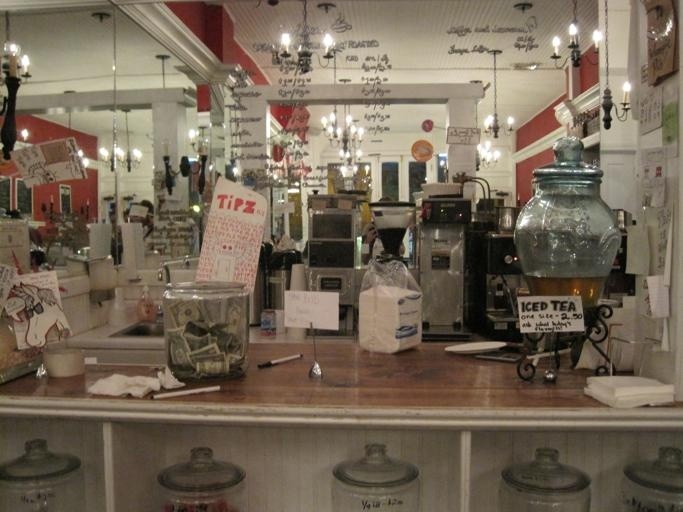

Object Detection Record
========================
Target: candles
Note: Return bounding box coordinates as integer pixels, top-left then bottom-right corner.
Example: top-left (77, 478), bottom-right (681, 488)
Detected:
top-left (9, 55), bottom-right (18, 77)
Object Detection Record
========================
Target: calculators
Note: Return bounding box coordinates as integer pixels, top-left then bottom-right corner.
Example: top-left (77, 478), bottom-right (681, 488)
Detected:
top-left (476, 351), bottom-right (521, 363)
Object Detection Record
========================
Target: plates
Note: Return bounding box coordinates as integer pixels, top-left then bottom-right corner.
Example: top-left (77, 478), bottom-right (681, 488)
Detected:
top-left (444, 342), bottom-right (507, 355)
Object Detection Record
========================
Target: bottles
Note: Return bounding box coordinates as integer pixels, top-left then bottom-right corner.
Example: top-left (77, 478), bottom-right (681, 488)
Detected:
top-left (623, 445), bottom-right (683, 512)
top-left (501, 447), bottom-right (594, 512)
top-left (153, 445), bottom-right (247, 512)
top-left (332, 442), bottom-right (422, 512)
top-left (163, 280), bottom-right (249, 383)
top-left (0, 440), bottom-right (88, 511)
top-left (513, 135), bottom-right (622, 312)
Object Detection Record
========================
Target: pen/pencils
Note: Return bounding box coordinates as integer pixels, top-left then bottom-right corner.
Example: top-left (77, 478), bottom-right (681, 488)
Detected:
top-left (150, 384), bottom-right (225, 400)
top-left (257, 353), bottom-right (303, 368)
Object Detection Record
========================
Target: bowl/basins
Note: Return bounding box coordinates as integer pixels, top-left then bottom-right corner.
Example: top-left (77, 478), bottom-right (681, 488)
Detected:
top-left (42, 350), bottom-right (87, 379)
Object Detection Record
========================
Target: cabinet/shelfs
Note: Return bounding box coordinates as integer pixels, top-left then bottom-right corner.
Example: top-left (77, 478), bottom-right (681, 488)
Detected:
top-left (0, 341), bottom-right (683, 511)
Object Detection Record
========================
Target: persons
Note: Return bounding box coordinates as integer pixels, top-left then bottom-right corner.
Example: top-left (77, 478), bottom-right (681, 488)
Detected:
top-left (359, 196), bottom-right (406, 264)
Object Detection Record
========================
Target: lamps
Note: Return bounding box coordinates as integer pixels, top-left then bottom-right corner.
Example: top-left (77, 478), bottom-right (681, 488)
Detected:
top-left (271, 1), bottom-right (335, 74)
top-left (1, 10), bottom-right (33, 85)
top-left (550, 0), bottom-right (605, 71)
top-left (320, 102), bottom-right (365, 168)
top-left (471, 48), bottom-right (515, 168)
top-left (600, 0), bottom-right (633, 130)
top-left (181, 126), bottom-right (220, 193)
top-left (99, 107), bottom-right (140, 172)
